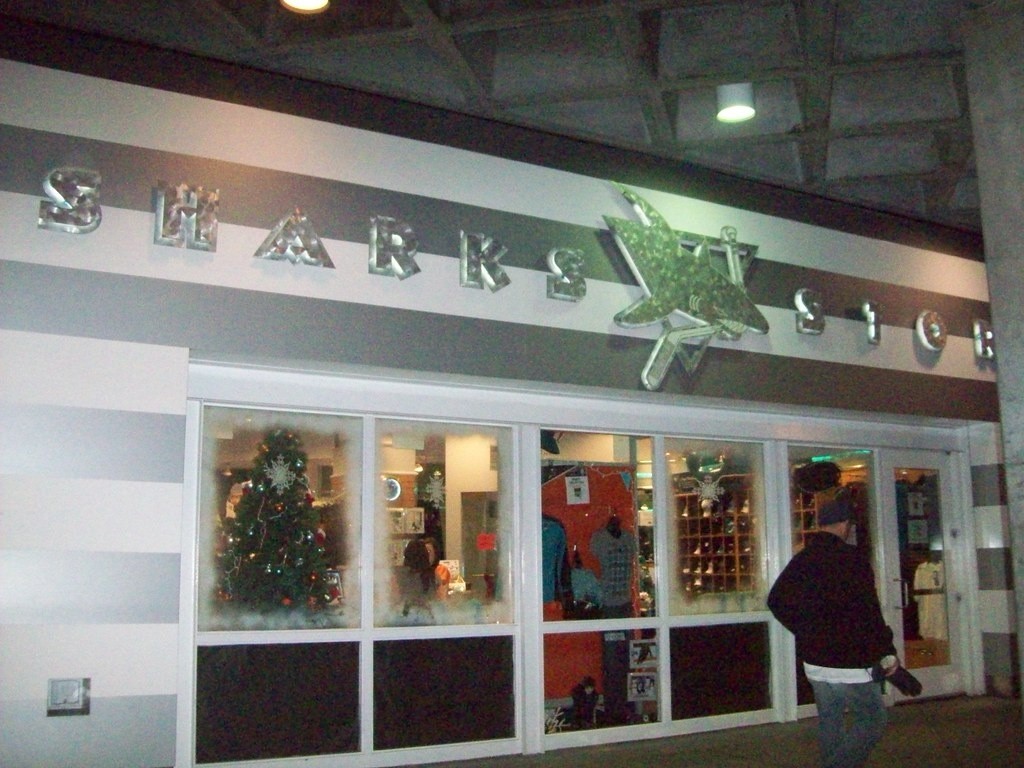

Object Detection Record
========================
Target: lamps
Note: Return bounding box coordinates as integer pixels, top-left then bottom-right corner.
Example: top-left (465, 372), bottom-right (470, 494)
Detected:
top-left (415, 464), bottom-right (423, 472)
top-left (717, 81), bottom-right (757, 123)
top-left (324, 570), bottom-right (346, 598)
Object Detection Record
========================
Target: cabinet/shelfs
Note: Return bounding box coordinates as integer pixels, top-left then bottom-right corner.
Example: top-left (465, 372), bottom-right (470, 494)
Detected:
top-left (675, 483), bottom-right (843, 608)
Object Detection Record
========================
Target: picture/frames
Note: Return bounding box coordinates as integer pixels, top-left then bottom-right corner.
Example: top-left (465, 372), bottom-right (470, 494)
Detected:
top-left (406, 508), bottom-right (425, 534)
top-left (907, 492), bottom-right (925, 516)
top-left (630, 639), bottom-right (657, 669)
top-left (907, 520), bottom-right (929, 543)
top-left (385, 508), bottom-right (405, 534)
top-left (387, 539), bottom-right (405, 567)
top-left (383, 477), bottom-right (403, 502)
top-left (627, 672), bottom-right (657, 702)
top-left (564, 476), bottom-right (590, 505)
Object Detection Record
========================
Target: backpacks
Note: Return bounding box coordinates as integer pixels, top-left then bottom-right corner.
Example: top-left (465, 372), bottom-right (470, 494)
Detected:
top-left (767, 543), bottom-right (851, 639)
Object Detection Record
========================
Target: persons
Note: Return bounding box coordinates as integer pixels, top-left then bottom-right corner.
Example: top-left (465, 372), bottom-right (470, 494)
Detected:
top-left (590, 514), bottom-right (638, 712)
top-left (912, 544), bottom-right (952, 663)
top-left (399, 538), bottom-right (450, 614)
top-left (766, 498), bottom-right (901, 768)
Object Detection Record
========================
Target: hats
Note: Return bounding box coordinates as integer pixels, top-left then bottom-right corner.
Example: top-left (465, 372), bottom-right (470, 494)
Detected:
top-left (816, 501), bottom-right (848, 525)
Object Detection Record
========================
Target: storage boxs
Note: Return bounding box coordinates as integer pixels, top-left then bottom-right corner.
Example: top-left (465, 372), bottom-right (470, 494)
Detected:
top-left (439, 559), bottom-right (466, 592)
top-left (638, 510), bottom-right (653, 527)
